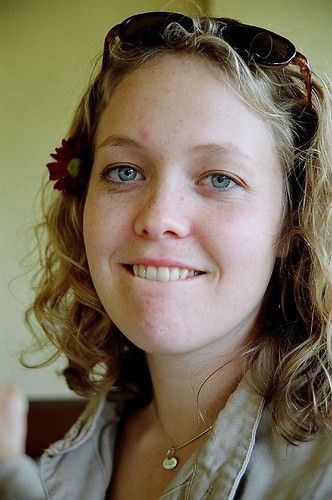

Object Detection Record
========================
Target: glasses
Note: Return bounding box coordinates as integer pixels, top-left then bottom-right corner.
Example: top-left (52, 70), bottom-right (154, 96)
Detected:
top-left (103, 10), bottom-right (313, 120)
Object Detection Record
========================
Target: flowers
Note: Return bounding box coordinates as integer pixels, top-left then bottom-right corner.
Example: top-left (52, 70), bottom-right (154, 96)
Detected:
top-left (47, 136), bottom-right (92, 197)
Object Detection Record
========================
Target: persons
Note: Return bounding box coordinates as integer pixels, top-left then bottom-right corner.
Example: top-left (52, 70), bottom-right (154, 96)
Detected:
top-left (1, 11), bottom-right (331, 497)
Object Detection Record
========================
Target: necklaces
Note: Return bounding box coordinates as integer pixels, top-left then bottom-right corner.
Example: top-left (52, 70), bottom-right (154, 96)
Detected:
top-left (146, 386), bottom-right (222, 471)
